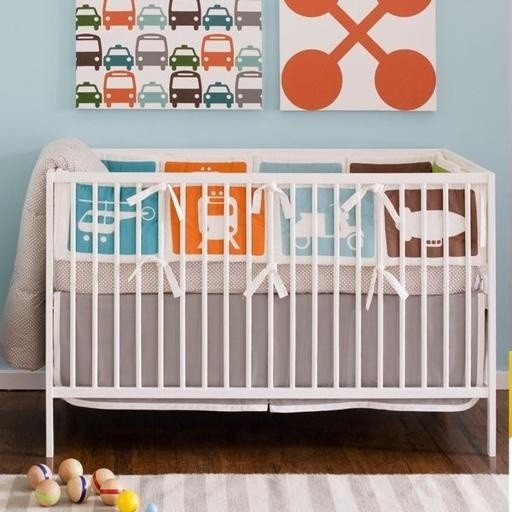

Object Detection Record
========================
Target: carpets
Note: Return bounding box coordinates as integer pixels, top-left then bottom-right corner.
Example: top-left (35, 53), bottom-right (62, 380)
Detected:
top-left (0, 473), bottom-right (510, 512)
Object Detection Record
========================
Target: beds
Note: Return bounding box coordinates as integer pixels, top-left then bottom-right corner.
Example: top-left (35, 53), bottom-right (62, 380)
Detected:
top-left (44, 146), bottom-right (496, 458)
